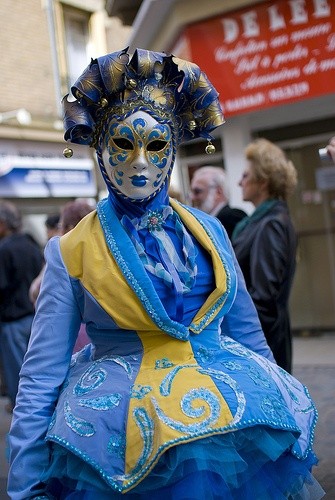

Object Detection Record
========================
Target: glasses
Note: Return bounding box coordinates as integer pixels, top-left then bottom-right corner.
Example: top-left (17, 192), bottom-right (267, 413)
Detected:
top-left (194, 182), bottom-right (217, 195)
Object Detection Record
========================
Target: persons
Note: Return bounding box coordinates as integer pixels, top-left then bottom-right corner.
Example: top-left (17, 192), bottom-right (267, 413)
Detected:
top-left (0, 199), bottom-right (95, 414)
top-left (231, 137), bottom-right (299, 374)
top-left (7, 45), bottom-right (326, 500)
top-left (189, 165), bottom-right (248, 240)
